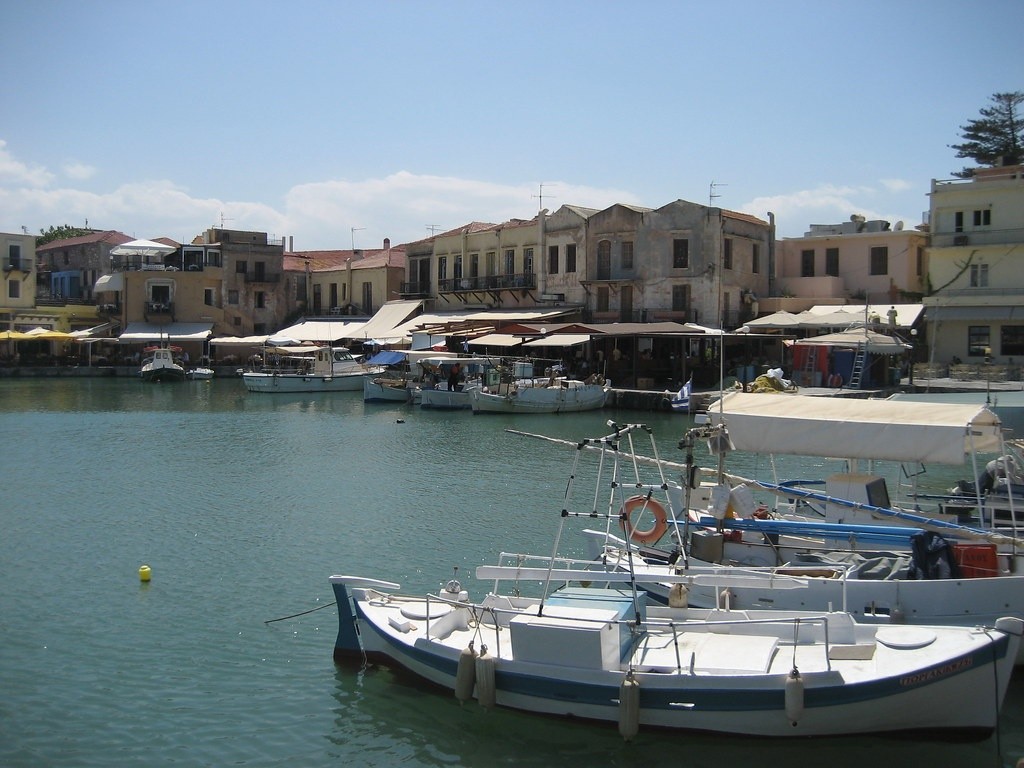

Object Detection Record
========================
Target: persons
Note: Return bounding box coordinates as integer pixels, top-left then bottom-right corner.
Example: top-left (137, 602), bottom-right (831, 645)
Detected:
top-left (642, 348), bottom-right (655, 361)
top-left (596, 345), bottom-right (622, 374)
top-left (102, 345), bottom-right (140, 365)
top-left (886, 305), bottom-right (897, 331)
top-left (868, 311), bottom-right (881, 333)
top-left (173, 351), bottom-right (190, 367)
top-left (448, 363), bottom-right (461, 392)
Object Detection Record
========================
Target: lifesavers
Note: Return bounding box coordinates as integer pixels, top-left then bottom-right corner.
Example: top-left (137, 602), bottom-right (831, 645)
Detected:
top-left (619, 495), bottom-right (667, 543)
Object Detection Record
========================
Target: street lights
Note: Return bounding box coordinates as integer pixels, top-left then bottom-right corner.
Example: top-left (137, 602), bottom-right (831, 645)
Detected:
top-left (909, 328), bottom-right (918, 383)
top-left (984, 347), bottom-right (992, 404)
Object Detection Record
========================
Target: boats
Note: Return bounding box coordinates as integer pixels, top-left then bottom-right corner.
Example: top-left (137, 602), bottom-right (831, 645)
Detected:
top-left (136, 327), bottom-right (184, 383)
top-left (185, 368), bottom-right (215, 380)
top-left (240, 341), bottom-right (613, 416)
top-left (325, 416), bottom-right (1024, 742)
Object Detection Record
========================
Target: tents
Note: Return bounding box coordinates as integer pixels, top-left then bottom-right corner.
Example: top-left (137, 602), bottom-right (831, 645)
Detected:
top-left (208, 335), bottom-right (269, 372)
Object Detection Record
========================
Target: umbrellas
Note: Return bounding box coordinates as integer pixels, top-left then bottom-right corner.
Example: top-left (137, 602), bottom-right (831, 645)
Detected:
top-left (110, 239), bottom-right (177, 274)
top-left (0, 326), bottom-right (79, 367)
top-left (743, 306), bottom-right (901, 366)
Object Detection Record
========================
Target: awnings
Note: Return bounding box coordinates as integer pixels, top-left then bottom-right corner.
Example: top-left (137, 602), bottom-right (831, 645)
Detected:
top-left (521, 334), bottom-right (590, 348)
top-left (465, 307), bottom-right (580, 321)
top-left (810, 304), bottom-right (927, 331)
top-left (416, 356), bottom-right (501, 366)
top-left (93, 272), bottom-right (124, 293)
top-left (460, 333), bottom-right (533, 347)
top-left (362, 350), bottom-right (408, 366)
top-left (76, 337), bottom-right (118, 344)
top-left (518, 322), bottom-right (705, 336)
top-left (118, 322), bottom-right (215, 342)
top-left (265, 298), bottom-right (485, 345)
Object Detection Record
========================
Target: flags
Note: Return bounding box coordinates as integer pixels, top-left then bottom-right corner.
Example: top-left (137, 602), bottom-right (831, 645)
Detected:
top-left (671, 377), bottom-right (691, 411)
